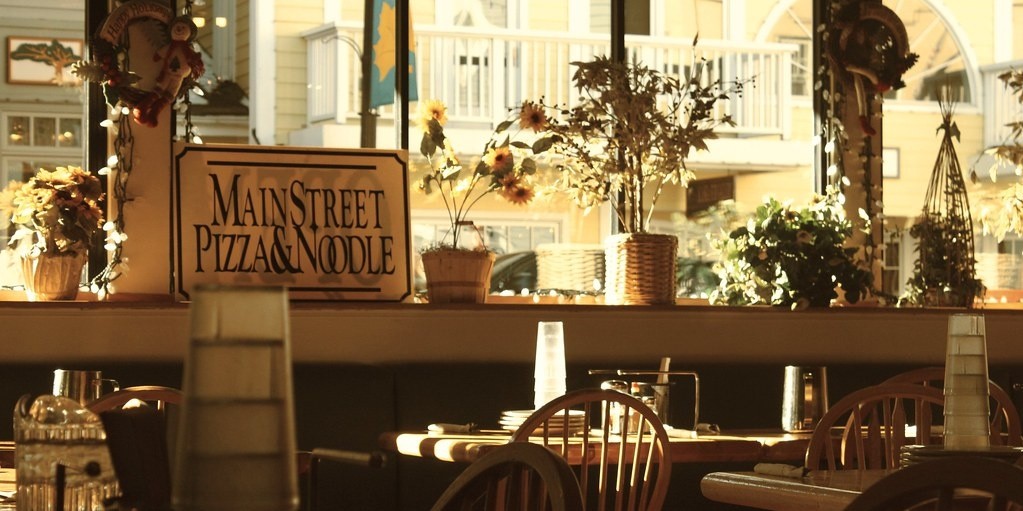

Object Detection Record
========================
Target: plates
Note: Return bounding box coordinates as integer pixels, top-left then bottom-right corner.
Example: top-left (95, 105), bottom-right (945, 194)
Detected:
top-left (498, 410), bottom-right (586, 437)
top-left (897, 444), bottom-right (1023, 471)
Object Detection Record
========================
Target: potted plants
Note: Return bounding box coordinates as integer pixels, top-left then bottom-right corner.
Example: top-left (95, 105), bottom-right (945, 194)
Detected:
top-left (533, 32), bottom-right (756, 304)
top-left (898, 89), bottom-right (987, 307)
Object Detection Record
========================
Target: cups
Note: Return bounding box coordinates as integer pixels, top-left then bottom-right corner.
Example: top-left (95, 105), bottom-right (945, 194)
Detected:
top-left (52, 368), bottom-right (120, 403)
top-left (532, 321), bottom-right (567, 410)
top-left (780, 365), bottom-right (828, 433)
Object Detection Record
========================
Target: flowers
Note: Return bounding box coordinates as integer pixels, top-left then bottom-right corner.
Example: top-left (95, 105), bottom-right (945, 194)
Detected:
top-left (3, 165), bottom-right (106, 253)
top-left (419, 101), bottom-right (549, 248)
top-left (708, 194), bottom-right (891, 311)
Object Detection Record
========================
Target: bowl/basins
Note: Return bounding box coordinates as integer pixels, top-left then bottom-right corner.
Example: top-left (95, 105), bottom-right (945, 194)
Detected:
top-left (942, 310), bottom-right (991, 449)
top-left (600, 380), bottom-right (670, 433)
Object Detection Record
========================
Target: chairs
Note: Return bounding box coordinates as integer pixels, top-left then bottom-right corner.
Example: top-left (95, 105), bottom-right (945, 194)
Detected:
top-left (841, 366), bottom-right (1020, 469)
top-left (804, 387), bottom-right (943, 470)
top-left (429, 441), bottom-right (582, 511)
top-left (86, 386), bottom-right (182, 415)
top-left (497, 389), bottom-right (670, 511)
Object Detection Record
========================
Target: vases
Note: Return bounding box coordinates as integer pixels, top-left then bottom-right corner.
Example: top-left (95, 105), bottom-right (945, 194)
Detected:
top-left (18, 255), bottom-right (90, 299)
top-left (420, 250), bottom-right (494, 305)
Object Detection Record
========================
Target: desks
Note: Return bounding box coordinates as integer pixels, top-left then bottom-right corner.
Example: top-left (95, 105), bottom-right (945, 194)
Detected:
top-left (765, 427), bottom-right (945, 459)
top-left (375, 432), bottom-right (761, 467)
top-left (701, 472), bottom-right (991, 511)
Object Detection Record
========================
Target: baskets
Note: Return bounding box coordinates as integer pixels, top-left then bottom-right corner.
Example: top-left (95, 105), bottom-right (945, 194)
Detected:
top-left (603, 234), bottom-right (677, 304)
